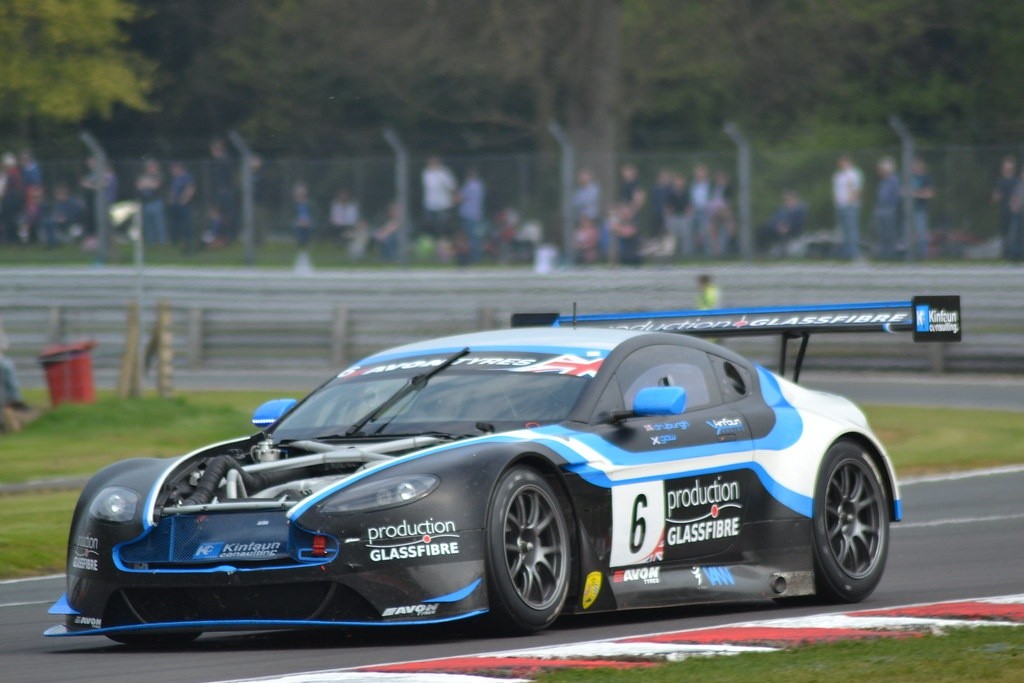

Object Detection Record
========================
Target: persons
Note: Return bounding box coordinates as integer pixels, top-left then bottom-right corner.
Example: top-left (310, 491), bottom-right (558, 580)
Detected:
top-left (759, 188), bottom-right (809, 258)
top-left (870, 155), bottom-right (901, 257)
top-left (906, 156), bottom-right (936, 264)
top-left (697, 273), bottom-right (721, 344)
top-left (0, 334), bottom-right (42, 432)
top-left (421, 152), bottom-right (458, 233)
top-left (834, 156), bottom-right (864, 258)
top-left (572, 161), bottom-right (737, 267)
top-left (0, 136), bottom-right (400, 267)
top-left (994, 157), bottom-right (1024, 267)
top-left (451, 161), bottom-right (485, 265)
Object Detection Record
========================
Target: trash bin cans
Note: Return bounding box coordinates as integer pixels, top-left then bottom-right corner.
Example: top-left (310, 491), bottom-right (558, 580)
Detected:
top-left (41, 340), bottom-right (95, 409)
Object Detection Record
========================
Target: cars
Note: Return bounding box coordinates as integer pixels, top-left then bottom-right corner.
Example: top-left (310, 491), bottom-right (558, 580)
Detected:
top-left (41, 294), bottom-right (962, 647)
